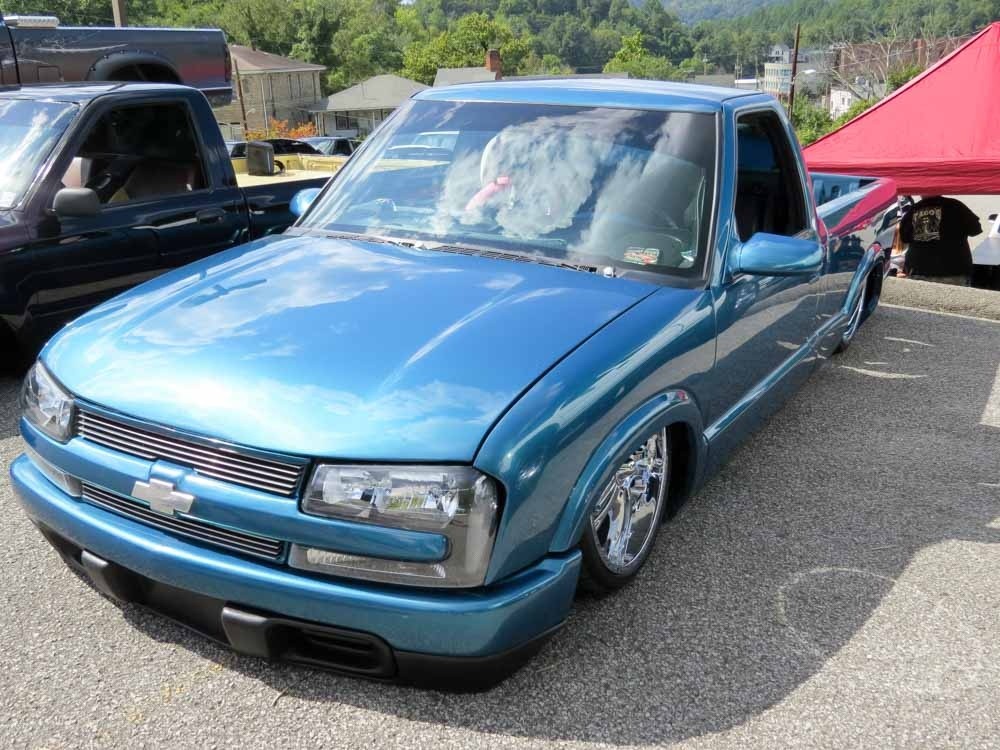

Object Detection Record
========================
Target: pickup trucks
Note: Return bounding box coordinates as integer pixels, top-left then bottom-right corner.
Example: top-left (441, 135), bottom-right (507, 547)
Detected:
top-left (0, 80), bottom-right (455, 388)
top-left (0, 10), bottom-right (238, 117)
top-left (8, 73), bottom-right (903, 694)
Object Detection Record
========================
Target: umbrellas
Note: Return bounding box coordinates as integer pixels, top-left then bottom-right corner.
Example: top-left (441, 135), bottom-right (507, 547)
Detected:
top-left (801, 19), bottom-right (1000, 241)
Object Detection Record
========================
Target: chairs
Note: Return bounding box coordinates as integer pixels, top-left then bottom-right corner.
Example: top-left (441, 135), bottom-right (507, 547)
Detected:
top-left (104, 133), bottom-right (200, 203)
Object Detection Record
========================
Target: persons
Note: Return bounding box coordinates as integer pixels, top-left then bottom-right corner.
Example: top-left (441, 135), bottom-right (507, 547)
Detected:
top-left (621, 157), bottom-right (689, 232)
top-left (84, 119), bottom-right (164, 208)
top-left (898, 191), bottom-right (983, 289)
top-left (458, 134), bottom-right (559, 226)
top-left (827, 233), bottom-right (864, 272)
